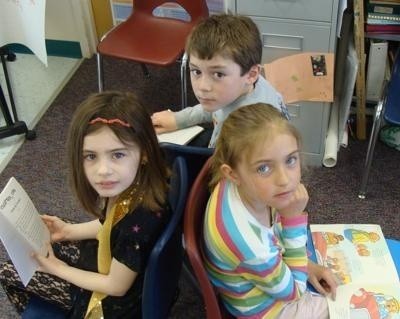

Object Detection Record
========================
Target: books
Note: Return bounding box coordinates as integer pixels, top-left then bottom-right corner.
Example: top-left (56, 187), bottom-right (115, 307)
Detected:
top-left (310, 224), bottom-right (400, 319)
top-left (0, 177), bottom-right (50, 287)
top-left (156, 109), bottom-right (204, 145)
top-left (366, 12), bottom-right (400, 35)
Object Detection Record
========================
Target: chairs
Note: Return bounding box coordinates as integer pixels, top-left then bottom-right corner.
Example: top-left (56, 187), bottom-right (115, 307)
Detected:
top-left (155, 143), bottom-right (217, 213)
top-left (183, 156), bottom-right (232, 318)
top-left (23, 156), bottom-right (188, 317)
top-left (96, 1), bottom-right (210, 115)
top-left (357, 69), bottom-right (400, 200)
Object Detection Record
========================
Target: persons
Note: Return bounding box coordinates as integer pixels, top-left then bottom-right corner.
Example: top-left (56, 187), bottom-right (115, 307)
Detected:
top-left (150, 14), bottom-right (290, 149)
top-left (202, 103), bottom-right (337, 319)
top-left (0, 92), bottom-right (175, 319)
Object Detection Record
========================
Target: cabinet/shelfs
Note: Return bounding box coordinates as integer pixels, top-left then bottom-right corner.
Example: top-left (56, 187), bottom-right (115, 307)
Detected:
top-left (230, 2), bottom-right (339, 168)
